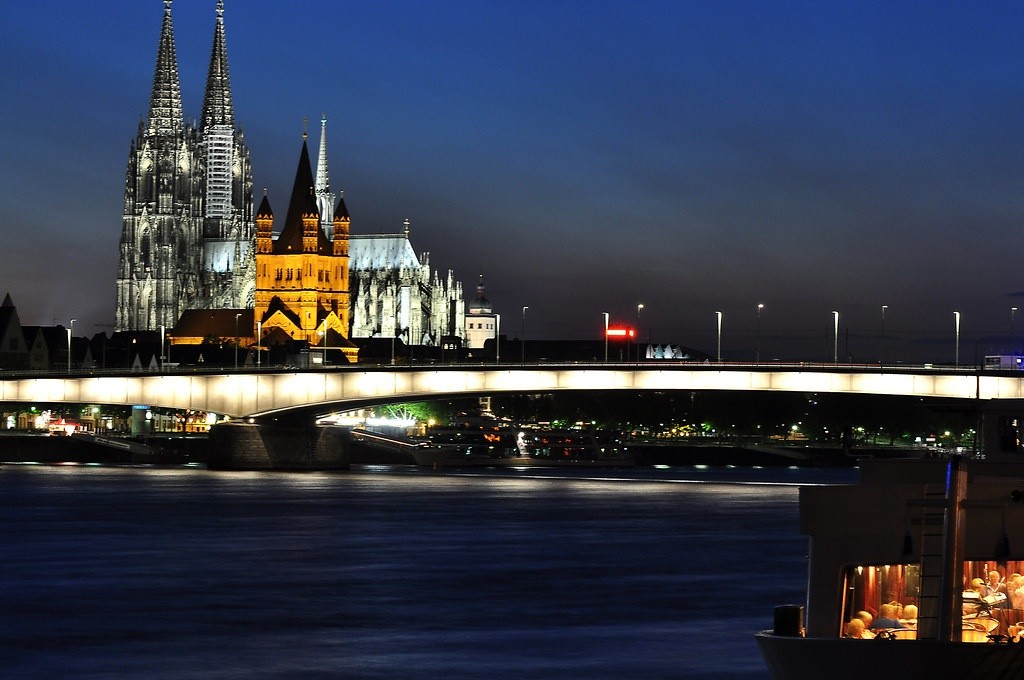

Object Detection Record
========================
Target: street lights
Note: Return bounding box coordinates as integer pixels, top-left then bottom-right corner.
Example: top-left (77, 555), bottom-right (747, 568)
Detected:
top-left (320, 318), bottom-right (328, 366)
top-left (494, 313), bottom-right (500, 364)
top-left (521, 306), bottom-right (530, 364)
top-left (256, 320), bottom-right (263, 366)
top-left (953, 311), bottom-right (960, 367)
top-left (65, 328), bottom-right (71, 372)
top-left (1010, 307), bottom-right (1017, 356)
top-left (234, 313), bottom-right (243, 368)
top-left (755, 304), bottom-right (764, 365)
top-left (158, 324), bottom-right (165, 372)
top-left (601, 312), bottom-right (609, 362)
top-left (636, 304), bottom-right (644, 369)
top-left (831, 310), bottom-right (839, 364)
top-left (715, 311), bottom-right (722, 363)
top-left (880, 305), bottom-right (888, 336)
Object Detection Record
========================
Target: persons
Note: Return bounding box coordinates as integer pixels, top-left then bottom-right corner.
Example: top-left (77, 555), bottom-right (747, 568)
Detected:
top-left (961, 570), bottom-right (1024, 643)
top-left (841, 600), bottom-right (918, 640)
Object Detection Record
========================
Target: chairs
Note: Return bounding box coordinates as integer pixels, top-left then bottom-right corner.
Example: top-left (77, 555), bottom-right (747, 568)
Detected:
top-left (870, 614), bottom-right (1000, 643)
